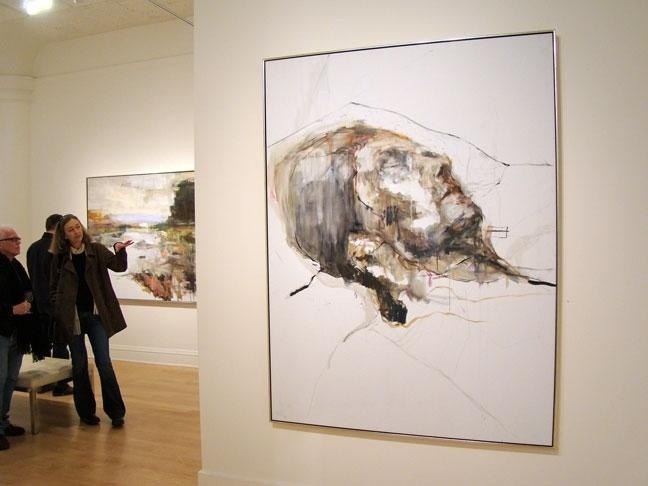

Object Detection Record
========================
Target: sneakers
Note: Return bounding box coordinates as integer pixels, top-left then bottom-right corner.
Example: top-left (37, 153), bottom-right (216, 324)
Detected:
top-left (0, 434), bottom-right (9, 451)
top-left (1, 420), bottom-right (26, 436)
top-left (51, 383), bottom-right (73, 397)
top-left (38, 383), bottom-right (54, 393)
top-left (79, 414), bottom-right (101, 423)
top-left (111, 419), bottom-right (124, 426)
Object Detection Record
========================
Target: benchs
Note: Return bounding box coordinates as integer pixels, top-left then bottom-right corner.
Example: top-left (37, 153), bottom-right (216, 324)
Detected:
top-left (15, 353), bottom-right (95, 434)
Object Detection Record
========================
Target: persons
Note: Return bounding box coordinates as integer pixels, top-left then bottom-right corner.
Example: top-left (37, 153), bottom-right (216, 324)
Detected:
top-left (0, 226), bottom-right (30, 450)
top-left (274, 124), bottom-right (553, 444)
top-left (45, 215), bottom-right (134, 426)
top-left (27, 213), bottom-right (75, 396)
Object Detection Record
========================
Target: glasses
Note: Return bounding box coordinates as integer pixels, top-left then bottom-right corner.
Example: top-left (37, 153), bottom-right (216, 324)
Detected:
top-left (0, 236), bottom-right (21, 242)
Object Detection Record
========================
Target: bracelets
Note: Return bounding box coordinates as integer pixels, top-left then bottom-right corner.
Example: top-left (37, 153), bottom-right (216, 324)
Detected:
top-left (114, 242), bottom-right (122, 247)
